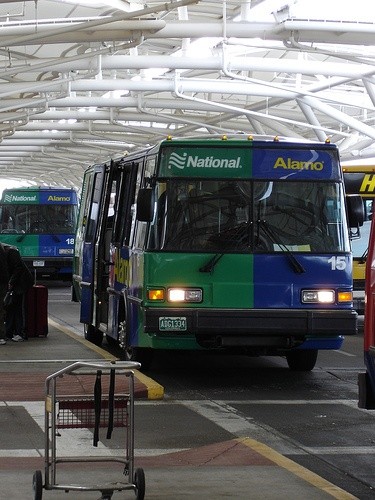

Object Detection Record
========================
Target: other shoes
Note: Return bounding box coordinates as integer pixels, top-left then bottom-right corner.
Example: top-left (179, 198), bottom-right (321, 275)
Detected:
top-left (12, 335), bottom-right (24, 342)
top-left (0, 339), bottom-right (6, 345)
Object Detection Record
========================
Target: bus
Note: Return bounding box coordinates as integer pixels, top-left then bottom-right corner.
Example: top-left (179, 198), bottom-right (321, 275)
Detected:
top-left (0, 186), bottom-right (80, 283)
top-left (70, 134), bottom-right (360, 384)
top-left (358, 191), bottom-right (375, 412)
top-left (337, 164), bottom-right (375, 313)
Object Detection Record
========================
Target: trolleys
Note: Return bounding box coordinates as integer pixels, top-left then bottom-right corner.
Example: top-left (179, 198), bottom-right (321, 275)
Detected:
top-left (32, 360), bottom-right (146, 500)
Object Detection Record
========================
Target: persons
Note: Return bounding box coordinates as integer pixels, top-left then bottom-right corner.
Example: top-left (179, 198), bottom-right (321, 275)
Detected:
top-left (0, 242), bottom-right (35, 345)
